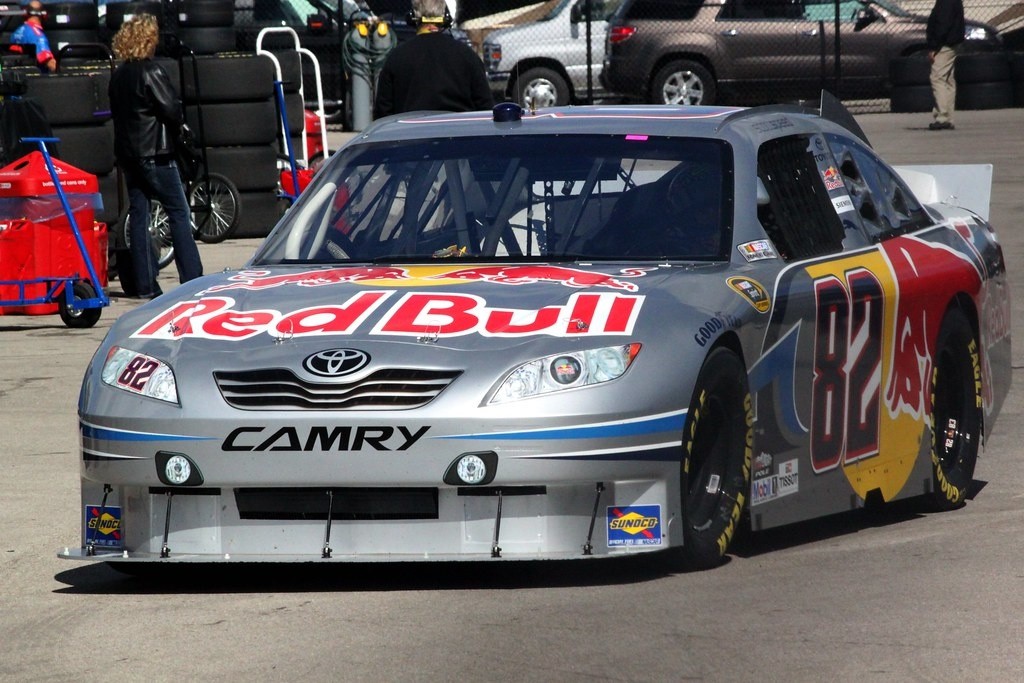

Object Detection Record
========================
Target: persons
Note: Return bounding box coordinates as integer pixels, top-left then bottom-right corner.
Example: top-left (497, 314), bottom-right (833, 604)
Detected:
top-left (926, 0), bottom-right (966, 130)
top-left (373, 0), bottom-right (496, 122)
top-left (110, 13), bottom-right (204, 299)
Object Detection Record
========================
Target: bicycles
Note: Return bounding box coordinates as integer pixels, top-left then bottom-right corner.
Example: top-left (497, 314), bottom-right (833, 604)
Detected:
top-left (119, 123), bottom-right (242, 270)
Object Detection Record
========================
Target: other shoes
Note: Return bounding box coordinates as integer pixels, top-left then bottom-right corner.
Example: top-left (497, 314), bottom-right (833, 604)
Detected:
top-left (929, 121), bottom-right (952, 130)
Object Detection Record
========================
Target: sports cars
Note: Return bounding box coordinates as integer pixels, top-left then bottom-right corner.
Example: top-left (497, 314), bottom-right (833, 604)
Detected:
top-left (58, 90), bottom-right (1013, 578)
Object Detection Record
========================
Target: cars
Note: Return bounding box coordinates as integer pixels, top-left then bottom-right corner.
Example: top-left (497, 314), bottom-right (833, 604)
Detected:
top-left (484, 0), bottom-right (612, 107)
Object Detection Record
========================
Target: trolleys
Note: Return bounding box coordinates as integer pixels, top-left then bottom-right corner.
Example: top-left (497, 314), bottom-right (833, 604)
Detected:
top-left (257, 27), bottom-right (352, 236)
top-left (0, 138), bottom-right (108, 328)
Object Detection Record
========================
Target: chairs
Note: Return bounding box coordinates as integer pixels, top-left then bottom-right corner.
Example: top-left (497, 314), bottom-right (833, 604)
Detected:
top-left (575, 157), bottom-right (722, 256)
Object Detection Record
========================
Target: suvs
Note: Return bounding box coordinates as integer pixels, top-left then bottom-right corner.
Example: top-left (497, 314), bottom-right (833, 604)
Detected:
top-left (99, 0), bottom-right (471, 123)
top-left (598, 0), bottom-right (1005, 106)
